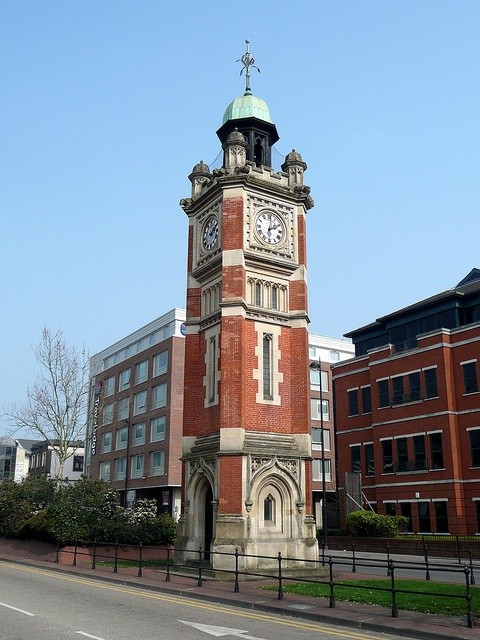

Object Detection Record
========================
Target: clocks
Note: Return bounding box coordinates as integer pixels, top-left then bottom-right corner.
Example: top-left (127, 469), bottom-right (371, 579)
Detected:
top-left (197, 210), bottom-right (221, 254)
top-left (253, 209), bottom-right (293, 251)
